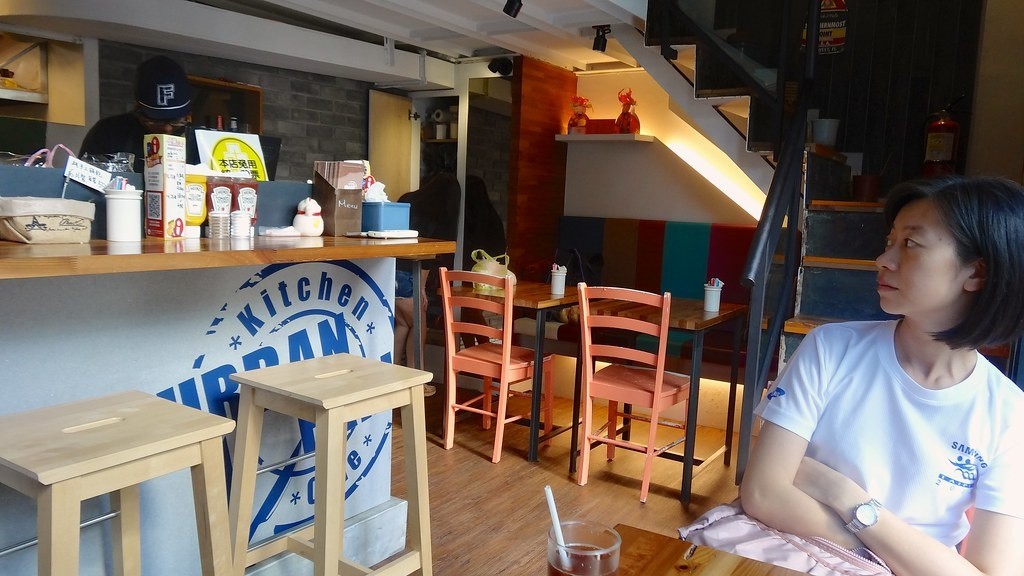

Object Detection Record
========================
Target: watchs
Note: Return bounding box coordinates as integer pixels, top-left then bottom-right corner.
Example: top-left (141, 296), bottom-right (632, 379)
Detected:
top-left (844, 498), bottom-right (883, 535)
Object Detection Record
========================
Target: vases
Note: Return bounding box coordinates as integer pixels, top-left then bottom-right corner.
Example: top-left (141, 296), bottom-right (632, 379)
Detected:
top-left (811, 118), bottom-right (840, 147)
top-left (852, 174), bottom-right (881, 203)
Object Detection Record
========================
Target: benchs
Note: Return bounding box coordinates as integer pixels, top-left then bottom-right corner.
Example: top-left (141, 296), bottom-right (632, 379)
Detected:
top-left (515, 309), bottom-right (779, 387)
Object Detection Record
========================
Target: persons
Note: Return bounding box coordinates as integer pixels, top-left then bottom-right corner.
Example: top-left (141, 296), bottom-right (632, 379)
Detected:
top-left (76, 61), bottom-right (217, 175)
top-left (388, 146), bottom-right (506, 394)
top-left (740, 174), bottom-right (1024, 576)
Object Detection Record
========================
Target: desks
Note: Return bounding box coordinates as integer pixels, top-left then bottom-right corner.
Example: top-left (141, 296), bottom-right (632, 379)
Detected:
top-left (437, 281), bottom-right (583, 463)
top-left (568, 298), bottom-right (747, 505)
top-left (603, 523), bottom-right (816, 576)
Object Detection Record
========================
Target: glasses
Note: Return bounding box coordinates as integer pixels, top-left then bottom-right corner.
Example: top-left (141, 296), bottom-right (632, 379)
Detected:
top-left (147, 111), bottom-right (188, 127)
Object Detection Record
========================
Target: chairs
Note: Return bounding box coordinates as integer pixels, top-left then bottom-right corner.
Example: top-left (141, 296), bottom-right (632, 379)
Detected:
top-left (577, 282), bottom-right (691, 503)
top-left (439, 267), bottom-right (555, 464)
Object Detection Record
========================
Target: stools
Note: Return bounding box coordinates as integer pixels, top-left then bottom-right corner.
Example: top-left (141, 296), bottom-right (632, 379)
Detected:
top-left (0, 390), bottom-right (236, 576)
top-left (229, 352), bottom-right (433, 576)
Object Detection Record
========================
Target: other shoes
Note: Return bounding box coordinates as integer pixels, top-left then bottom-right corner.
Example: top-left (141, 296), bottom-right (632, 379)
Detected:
top-left (423, 384), bottom-right (436, 397)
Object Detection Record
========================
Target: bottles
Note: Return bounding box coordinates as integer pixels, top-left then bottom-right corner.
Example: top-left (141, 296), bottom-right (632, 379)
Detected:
top-left (231, 117), bottom-right (238, 132)
top-left (217, 115), bottom-right (224, 131)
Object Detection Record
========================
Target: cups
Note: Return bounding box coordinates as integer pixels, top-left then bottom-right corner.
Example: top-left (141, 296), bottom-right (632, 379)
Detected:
top-left (209, 212), bottom-right (230, 240)
top-left (450, 119), bottom-right (458, 138)
top-left (703, 284), bottom-right (722, 312)
top-left (231, 212), bottom-right (252, 239)
top-left (436, 122), bottom-right (448, 139)
top-left (105, 189), bottom-right (144, 242)
top-left (239, 123), bottom-right (252, 133)
top-left (546, 521), bottom-right (621, 576)
top-left (551, 270), bottom-right (567, 295)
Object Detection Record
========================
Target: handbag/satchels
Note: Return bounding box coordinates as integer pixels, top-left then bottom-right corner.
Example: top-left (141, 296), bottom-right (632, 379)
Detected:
top-left (471, 249), bottom-right (518, 288)
top-left (677, 486), bottom-right (899, 576)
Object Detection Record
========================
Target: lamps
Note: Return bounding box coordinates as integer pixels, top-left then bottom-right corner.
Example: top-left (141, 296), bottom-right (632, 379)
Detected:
top-left (661, 44), bottom-right (677, 61)
top-left (503, 0), bottom-right (523, 18)
top-left (488, 58), bottom-right (514, 75)
top-left (592, 24), bottom-right (611, 53)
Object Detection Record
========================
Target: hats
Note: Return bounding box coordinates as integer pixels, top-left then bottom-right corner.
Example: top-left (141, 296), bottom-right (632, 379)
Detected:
top-left (134, 56), bottom-right (195, 121)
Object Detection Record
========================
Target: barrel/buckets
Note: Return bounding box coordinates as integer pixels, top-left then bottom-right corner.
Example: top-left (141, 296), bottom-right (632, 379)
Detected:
top-left (811, 118), bottom-right (839, 146)
top-left (853, 175), bottom-right (880, 202)
